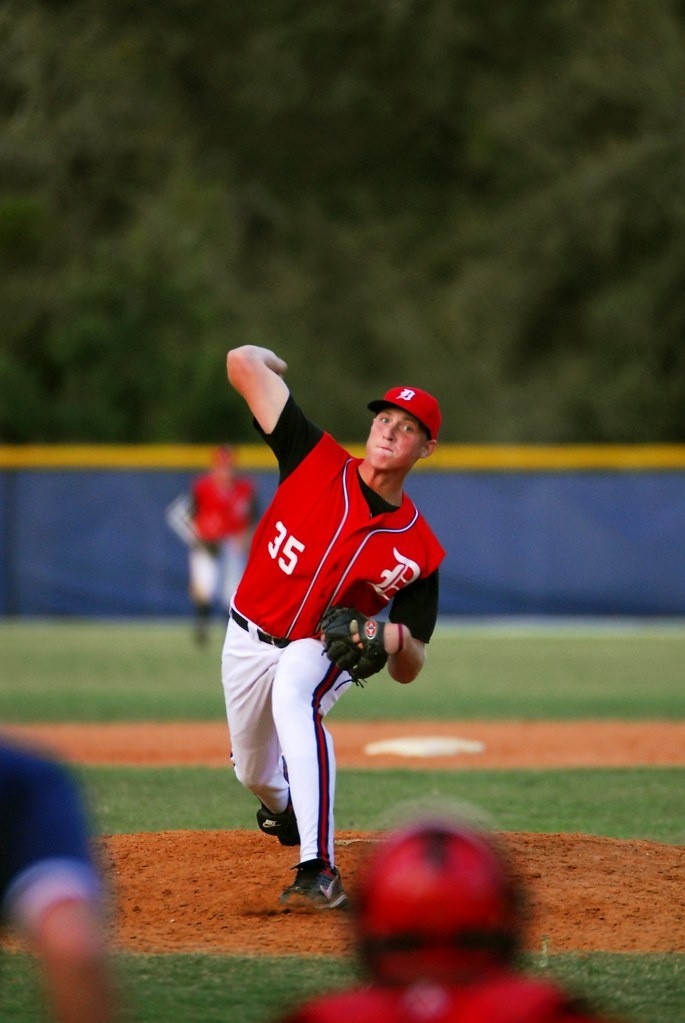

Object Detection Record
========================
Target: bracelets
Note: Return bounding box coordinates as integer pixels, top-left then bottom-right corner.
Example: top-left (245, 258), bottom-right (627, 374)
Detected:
top-left (391, 622), bottom-right (403, 659)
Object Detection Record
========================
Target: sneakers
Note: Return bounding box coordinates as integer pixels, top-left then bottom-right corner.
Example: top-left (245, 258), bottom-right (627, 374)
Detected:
top-left (279, 858), bottom-right (348, 909)
top-left (256, 756), bottom-right (300, 846)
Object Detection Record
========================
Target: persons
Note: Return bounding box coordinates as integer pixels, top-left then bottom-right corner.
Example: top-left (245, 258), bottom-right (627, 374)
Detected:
top-left (167, 444), bottom-right (259, 644)
top-left (284, 795), bottom-right (605, 1023)
top-left (221, 344), bottom-right (447, 908)
top-left (0, 744), bottom-right (116, 1023)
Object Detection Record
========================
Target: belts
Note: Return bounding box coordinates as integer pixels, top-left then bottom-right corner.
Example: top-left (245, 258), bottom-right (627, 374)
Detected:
top-left (231, 609), bottom-right (292, 649)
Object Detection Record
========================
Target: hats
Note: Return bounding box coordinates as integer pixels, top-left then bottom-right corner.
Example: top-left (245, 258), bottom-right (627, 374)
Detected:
top-left (368, 386), bottom-right (441, 440)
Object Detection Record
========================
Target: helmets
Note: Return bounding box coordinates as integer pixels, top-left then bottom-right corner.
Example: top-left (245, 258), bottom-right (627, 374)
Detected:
top-left (356, 821), bottom-right (511, 985)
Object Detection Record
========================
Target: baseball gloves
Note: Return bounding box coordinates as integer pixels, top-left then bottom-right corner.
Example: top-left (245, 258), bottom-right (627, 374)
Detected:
top-left (317, 605), bottom-right (388, 692)
